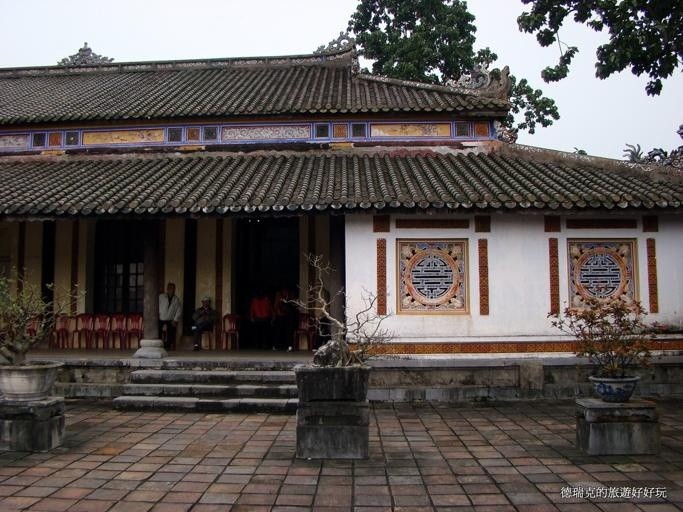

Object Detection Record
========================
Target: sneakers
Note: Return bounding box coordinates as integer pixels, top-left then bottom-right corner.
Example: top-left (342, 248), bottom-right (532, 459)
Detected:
top-left (194, 346), bottom-right (199, 352)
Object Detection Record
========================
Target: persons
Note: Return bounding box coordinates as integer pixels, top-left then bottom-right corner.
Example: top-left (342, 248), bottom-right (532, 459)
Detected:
top-left (312, 278), bottom-right (331, 354)
top-left (271, 286), bottom-right (297, 353)
top-left (190, 296), bottom-right (216, 352)
top-left (158, 281), bottom-right (182, 352)
top-left (249, 287), bottom-right (276, 353)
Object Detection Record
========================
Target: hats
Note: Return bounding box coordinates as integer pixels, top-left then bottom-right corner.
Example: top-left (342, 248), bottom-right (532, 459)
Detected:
top-left (202, 297), bottom-right (210, 301)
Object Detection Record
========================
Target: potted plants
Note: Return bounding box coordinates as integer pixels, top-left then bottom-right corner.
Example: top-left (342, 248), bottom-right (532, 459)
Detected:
top-left (0, 266), bottom-right (85, 400)
top-left (547, 292), bottom-right (667, 403)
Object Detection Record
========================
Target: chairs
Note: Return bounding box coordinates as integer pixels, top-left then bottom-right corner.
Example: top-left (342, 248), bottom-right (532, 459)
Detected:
top-left (293, 313), bottom-right (314, 351)
top-left (22, 313), bottom-right (241, 351)
